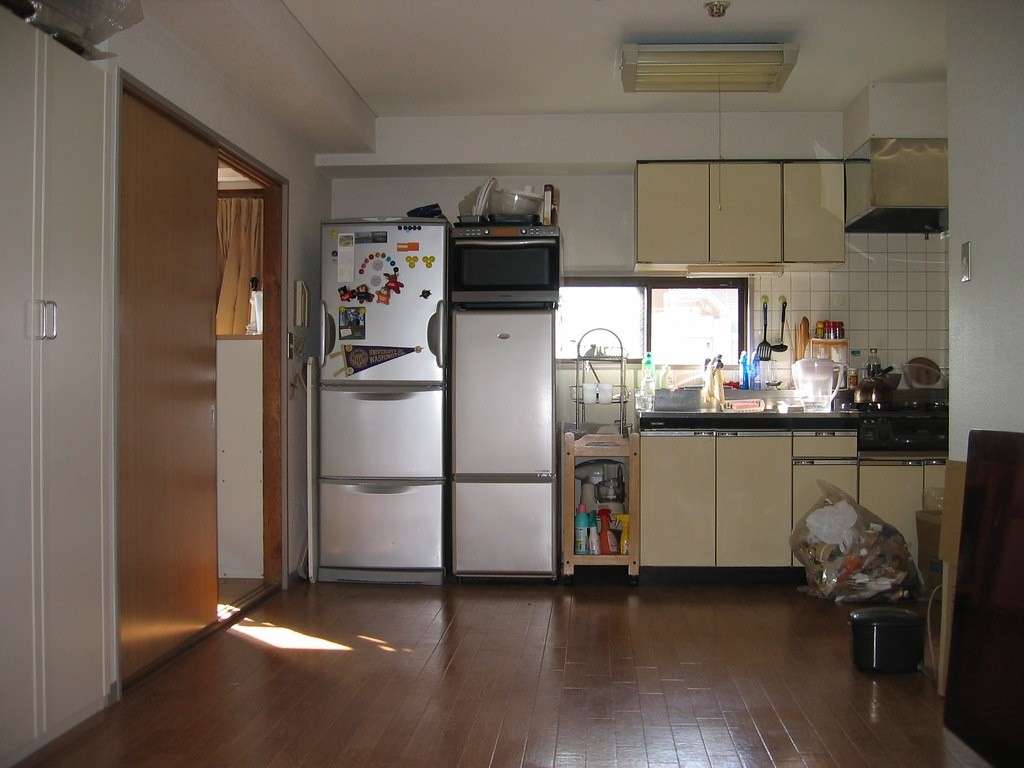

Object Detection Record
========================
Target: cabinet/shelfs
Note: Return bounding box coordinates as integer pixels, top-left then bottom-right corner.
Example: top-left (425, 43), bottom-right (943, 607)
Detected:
top-left (563, 328), bottom-right (640, 587)
top-left (633, 159), bottom-right (846, 272)
top-left (0, 5), bottom-right (120, 768)
top-left (639, 428), bottom-right (947, 588)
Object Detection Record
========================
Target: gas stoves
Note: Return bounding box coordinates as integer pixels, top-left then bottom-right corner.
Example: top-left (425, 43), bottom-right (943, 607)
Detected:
top-left (832, 387), bottom-right (949, 452)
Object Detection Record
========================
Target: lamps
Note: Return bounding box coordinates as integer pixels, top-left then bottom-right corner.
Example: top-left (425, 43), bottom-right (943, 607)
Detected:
top-left (618, 42), bottom-right (800, 92)
top-left (684, 267), bottom-right (784, 278)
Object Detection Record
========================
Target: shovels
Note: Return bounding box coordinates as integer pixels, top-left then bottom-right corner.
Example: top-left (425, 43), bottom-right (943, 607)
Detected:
top-left (756, 302), bottom-right (772, 361)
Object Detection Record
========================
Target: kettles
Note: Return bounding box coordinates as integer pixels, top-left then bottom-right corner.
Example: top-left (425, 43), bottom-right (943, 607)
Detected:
top-left (853, 366), bottom-right (895, 404)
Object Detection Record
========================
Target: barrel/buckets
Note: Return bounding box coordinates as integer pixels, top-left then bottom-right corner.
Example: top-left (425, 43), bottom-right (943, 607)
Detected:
top-left (849, 606), bottom-right (926, 674)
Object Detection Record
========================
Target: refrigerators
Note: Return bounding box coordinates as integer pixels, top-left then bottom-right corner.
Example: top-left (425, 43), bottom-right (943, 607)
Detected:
top-left (313, 216), bottom-right (450, 587)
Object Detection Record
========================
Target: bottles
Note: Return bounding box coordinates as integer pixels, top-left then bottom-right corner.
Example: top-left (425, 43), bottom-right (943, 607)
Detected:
top-left (847, 368), bottom-right (859, 390)
top-left (866, 348), bottom-right (881, 378)
top-left (738, 350), bottom-right (762, 391)
top-left (659, 365), bottom-right (675, 389)
top-left (815, 320), bottom-right (844, 340)
top-left (641, 352), bottom-right (656, 397)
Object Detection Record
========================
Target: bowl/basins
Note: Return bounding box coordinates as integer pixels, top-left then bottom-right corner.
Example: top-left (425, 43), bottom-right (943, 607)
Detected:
top-left (879, 373), bottom-right (901, 390)
top-left (488, 190), bottom-right (541, 218)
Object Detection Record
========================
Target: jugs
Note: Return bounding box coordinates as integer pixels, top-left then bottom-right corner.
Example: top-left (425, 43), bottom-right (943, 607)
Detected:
top-left (792, 357), bottom-right (844, 414)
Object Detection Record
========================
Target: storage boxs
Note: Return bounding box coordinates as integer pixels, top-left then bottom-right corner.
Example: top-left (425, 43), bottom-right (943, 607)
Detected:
top-left (916, 510), bottom-right (942, 601)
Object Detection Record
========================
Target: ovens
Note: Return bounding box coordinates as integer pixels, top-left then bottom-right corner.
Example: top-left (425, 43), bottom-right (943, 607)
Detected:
top-left (449, 228), bottom-right (564, 309)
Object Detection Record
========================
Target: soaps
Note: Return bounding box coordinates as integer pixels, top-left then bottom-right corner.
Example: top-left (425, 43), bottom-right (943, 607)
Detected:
top-left (735, 402), bottom-right (759, 409)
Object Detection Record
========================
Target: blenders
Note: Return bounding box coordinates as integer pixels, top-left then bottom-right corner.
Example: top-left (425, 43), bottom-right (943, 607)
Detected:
top-left (573, 458), bottom-right (625, 520)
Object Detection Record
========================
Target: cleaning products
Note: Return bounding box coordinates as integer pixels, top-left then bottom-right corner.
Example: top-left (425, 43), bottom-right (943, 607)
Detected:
top-left (700, 338), bottom-right (724, 406)
top-left (659, 361), bottom-right (674, 389)
top-left (574, 505), bottom-right (629, 555)
top-left (738, 351), bottom-right (761, 390)
top-left (641, 352), bottom-right (656, 388)
